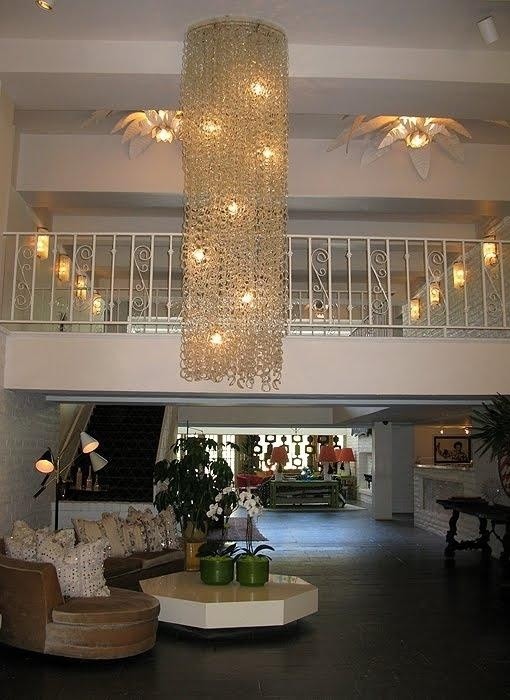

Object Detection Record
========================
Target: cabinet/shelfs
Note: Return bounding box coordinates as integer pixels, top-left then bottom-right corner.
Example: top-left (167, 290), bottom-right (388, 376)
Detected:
top-left (271, 480), bottom-right (339, 509)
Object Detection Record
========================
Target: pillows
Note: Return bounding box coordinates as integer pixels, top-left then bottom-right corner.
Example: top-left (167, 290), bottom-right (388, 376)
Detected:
top-left (127, 505), bottom-right (156, 552)
top-left (11, 518), bottom-right (75, 552)
top-left (102, 511), bottom-right (147, 552)
top-left (36, 539), bottom-right (109, 598)
top-left (5, 535), bottom-right (62, 570)
top-left (153, 504), bottom-right (179, 552)
top-left (70, 513), bottom-right (128, 560)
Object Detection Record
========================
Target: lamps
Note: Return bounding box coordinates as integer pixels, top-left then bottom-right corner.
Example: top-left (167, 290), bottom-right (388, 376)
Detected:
top-left (270, 447), bottom-right (290, 482)
top-left (33, 447), bottom-right (108, 499)
top-left (110, 109), bottom-right (184, 159)
top-left (410, 298), bottom-right (420, 321)
top-left (37, 227), bottom-right (49, 263)
top-left (337, 448), bottom-right (356, 478)
top-left (178, 16), bottom-right (289, 389)
top-left (476, 16), bottom-right (501, 50)
top-left (319, 446), bottom-right (337, 481)
top-left (56, 253), bottom-right (72, 280)
top-left (431, 281), bottom-right (441, 304)
top-left (35, 449), bottom-right (64, 483)
top-left (77, 275), bottom-right (88, 301)
top-left (453, 263), bottom-right (465, 290)
top-left (93, 293), bottom-right (102, 317)
top-left (325, 113), bottom-right (472, 181)
top-left (483, 236), bottom-right (500, 268)
top-left (33, 432), bottom-right (99, 486)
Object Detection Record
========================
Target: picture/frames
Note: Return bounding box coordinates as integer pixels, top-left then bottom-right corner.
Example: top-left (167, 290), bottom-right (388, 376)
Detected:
top-left (433, 436), bottom-right (473, 465)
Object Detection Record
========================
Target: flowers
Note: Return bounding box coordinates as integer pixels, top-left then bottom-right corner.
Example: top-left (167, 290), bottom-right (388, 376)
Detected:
top-left (234, 491), bottom-right (275, 561)
top-left (196, 486), bottom-right (235, 558)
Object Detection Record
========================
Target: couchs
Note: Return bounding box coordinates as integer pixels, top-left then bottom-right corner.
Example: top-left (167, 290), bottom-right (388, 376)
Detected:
top-left (0, 536), bottom-right (184, 670)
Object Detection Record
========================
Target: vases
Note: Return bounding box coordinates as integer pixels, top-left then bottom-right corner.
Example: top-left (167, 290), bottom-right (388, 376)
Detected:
top-left (236, 554), bottom-right (270, 587)
top-left (200, 556), bottom-right (234, 586)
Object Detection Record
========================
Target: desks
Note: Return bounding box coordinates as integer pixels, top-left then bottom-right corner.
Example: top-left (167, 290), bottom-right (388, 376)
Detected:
top-left (436, 498), bottom-right (510, 580)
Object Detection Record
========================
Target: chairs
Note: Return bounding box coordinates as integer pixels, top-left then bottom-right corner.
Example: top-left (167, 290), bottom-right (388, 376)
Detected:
top-left (237, 474), bottom-right (265, 509)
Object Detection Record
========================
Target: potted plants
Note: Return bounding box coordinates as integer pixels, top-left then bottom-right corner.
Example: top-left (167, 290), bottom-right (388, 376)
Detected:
top-left (470, 393), bottom-right (510, 499)
top-left (153, 437), bottom-right (232, 572)
